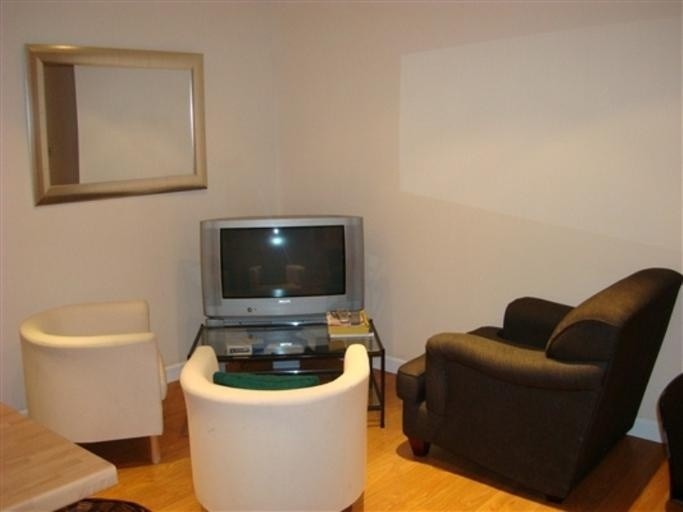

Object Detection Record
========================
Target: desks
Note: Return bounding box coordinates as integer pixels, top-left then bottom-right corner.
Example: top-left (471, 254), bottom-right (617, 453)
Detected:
top-left (0, 400), bottom-right (121, 511)
top-left (186, 319), bottom-right (386, 430)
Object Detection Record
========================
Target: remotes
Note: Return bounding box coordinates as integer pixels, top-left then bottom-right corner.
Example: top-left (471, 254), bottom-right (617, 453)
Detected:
top-left (336, 311), bottom-right (362, 325)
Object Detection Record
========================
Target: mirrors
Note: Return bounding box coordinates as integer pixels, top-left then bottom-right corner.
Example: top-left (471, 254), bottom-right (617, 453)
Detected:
top-left (21, 40), bottom-right (209, 209)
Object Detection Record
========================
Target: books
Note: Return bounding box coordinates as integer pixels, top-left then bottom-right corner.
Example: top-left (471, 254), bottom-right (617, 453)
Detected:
top-left (324, 306), bottom-right (369, 333)
top-left (327, 321), bottom-right (374, 340)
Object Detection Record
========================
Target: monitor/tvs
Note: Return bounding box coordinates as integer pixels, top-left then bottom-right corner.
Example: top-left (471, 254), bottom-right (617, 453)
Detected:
top-left (200, 214), bottom-right (366, 329)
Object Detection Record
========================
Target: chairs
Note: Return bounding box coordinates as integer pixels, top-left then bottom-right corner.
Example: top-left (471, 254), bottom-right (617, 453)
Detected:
top-left (394, 267), bottom-right (683, 504)
top-left (17, 303), bottom-right (167, 464)
top-left (178, 346), bottom-right (367, 510)
